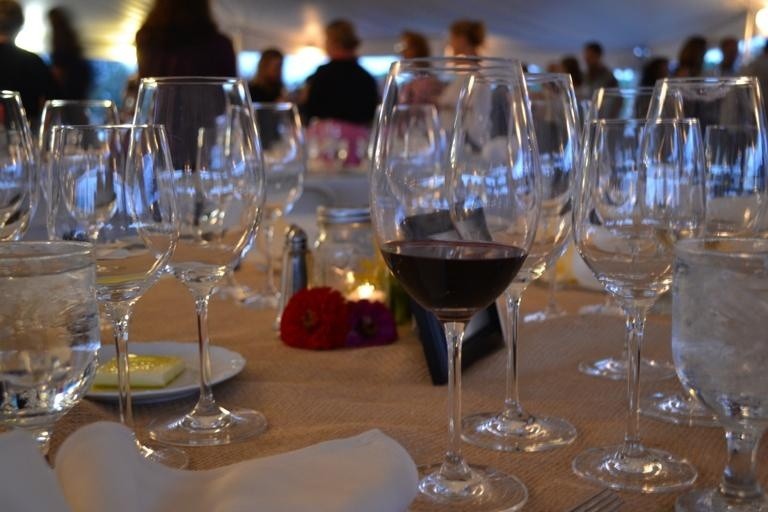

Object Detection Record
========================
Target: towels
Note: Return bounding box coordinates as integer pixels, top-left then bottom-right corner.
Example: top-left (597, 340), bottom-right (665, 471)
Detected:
top-left (0, 413), bottom-right (421, 512)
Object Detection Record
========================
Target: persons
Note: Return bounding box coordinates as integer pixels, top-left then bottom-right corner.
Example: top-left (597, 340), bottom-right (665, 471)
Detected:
top-left (2, 0), bottom-right (765, 168)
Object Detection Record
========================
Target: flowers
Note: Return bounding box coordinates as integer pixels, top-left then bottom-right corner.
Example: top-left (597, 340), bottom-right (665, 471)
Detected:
top-left (275, 284), bottom-right (345, 349)
top-left (339, 296), bottom-right (399, 347)
top-left (305, 117), bottom-right (368, 172)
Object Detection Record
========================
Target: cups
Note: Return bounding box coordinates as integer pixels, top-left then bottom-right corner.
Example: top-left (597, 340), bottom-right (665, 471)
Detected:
top-left (311, 119), bottom-right (369, 175)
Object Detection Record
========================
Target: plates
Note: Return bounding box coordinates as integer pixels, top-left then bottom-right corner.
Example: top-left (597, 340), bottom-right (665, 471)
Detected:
top-left (83, 340), bottom-right (248, 400)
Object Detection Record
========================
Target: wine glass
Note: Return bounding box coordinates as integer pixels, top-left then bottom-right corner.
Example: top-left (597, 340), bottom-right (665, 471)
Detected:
top-left (36, 97), bottom-right (124, 237)
top-left (586, 87), bottom-right (684, 227)
top-left (668, 236), bottom-right (768, 512)
top-left (0, 91), bottom-right (39, 237)
top-left (40, 123), bottom-right (191, 474)
top-left (0, 238), bottom-right (103, 465)
top-left (635, 75), bottom-right (768, 252)
top-left (447, 66), bottom-right (584, 454)
top-left (366, 104), bottom-right (452, 218)
top-left (569, 118), bottom-right (712, 496)
top-left (222, 101), bottom-right (307, 310)
top-left (127, 76), bottom-right (270, 450)
top-left (371, 56), bottom-right (542, 511)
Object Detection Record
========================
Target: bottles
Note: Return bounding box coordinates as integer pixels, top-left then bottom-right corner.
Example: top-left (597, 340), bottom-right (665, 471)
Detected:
top-left (317, 204), bottom-right (384, 295)
